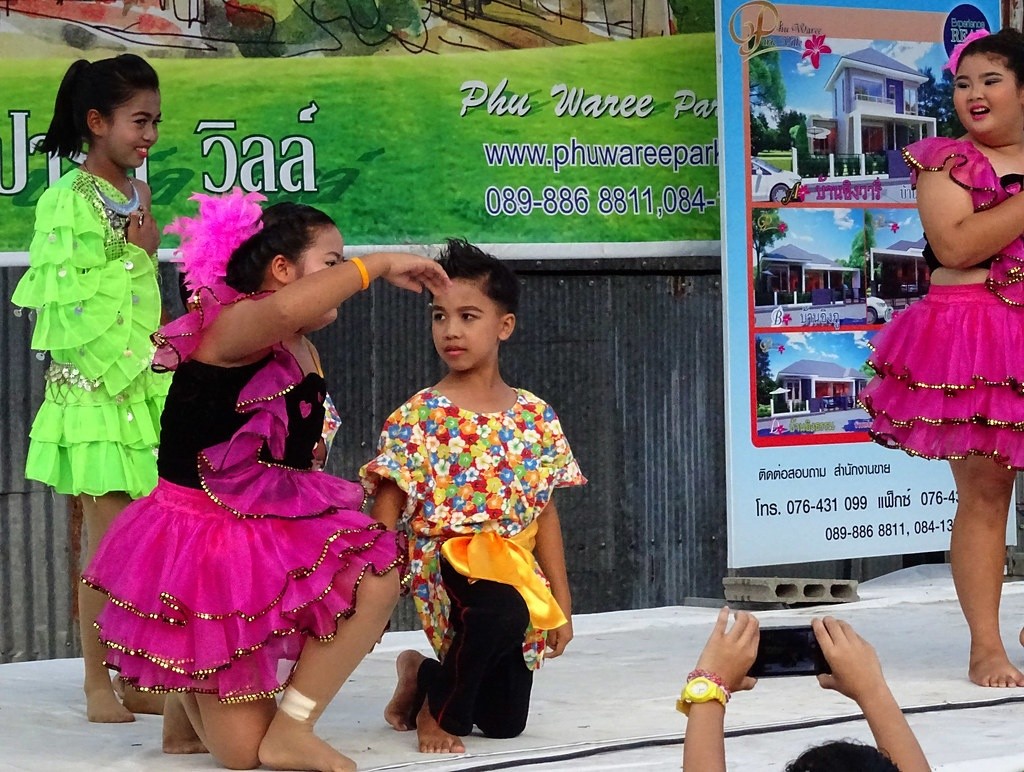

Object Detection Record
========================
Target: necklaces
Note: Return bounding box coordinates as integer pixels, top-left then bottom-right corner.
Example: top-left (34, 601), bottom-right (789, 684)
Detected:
top-left (84, 160), bottom-right (140, 227)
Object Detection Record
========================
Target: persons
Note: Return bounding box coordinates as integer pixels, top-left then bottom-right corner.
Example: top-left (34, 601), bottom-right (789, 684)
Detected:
top-left (81, 186), bottom-right (414, 772)
top-left (857, 27), bottom-right (1024, 686)
top-left (682, 605), bottom-right (932, 772)
top-left (10, 53), bottom-right (179, 722)
top-left (359, 237), bottom-right (589, 757)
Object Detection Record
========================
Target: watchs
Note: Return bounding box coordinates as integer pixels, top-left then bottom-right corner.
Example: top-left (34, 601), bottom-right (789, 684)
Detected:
top-left (675, 678), bottom-right (729, 716)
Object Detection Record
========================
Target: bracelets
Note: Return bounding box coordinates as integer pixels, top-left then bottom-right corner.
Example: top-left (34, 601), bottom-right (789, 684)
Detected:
top-left (688, 670), bottom-right (729, 696)
top-left (350, 256), bottom-right (370, 291)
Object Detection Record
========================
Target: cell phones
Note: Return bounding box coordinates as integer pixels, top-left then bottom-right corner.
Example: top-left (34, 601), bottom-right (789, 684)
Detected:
top-left (746, 626), bottom-right (832, 679)
